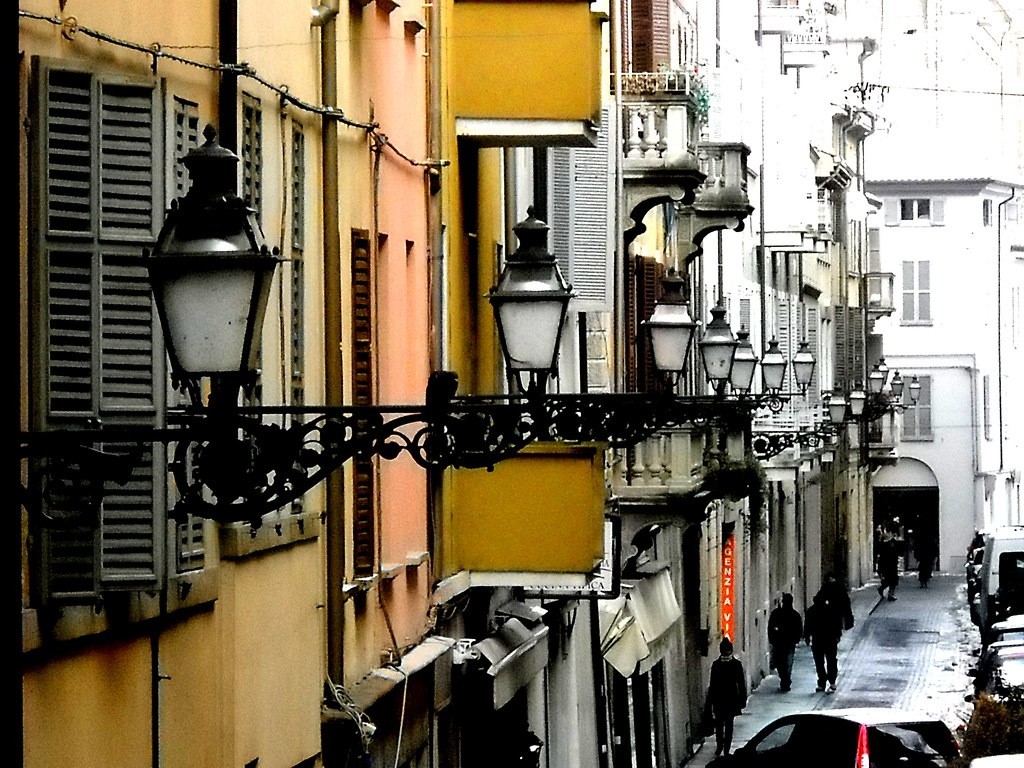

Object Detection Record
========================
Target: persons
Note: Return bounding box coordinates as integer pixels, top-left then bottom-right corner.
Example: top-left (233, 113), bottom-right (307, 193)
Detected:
top-left (915, 540), bottom-right (940, 589)
top-left (768, 593), bottom-right (803, 694)
top-left (804, 571), bottom-right (854, 692)
top-left (705, 637), bottom-right (747, 756)
top-left (877, 540), bottom-right (906, 601)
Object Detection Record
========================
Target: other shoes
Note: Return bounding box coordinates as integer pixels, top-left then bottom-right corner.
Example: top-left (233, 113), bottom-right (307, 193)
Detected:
top-left (816, 686), bottom-right (825, 692)
top-left (715, 745), bottom-right (723, 755)
top-left (878, 588), bottom-right (883, 596)
top-left (888, 596), bottom-right (897, 601)
top-left (829, 683), bottom-right (836, 690)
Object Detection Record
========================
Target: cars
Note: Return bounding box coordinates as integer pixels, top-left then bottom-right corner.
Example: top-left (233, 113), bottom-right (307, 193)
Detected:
top-left (706, 707), bottom-right (963, 768)
top-left (964, 528), bottom-right (1024, 703)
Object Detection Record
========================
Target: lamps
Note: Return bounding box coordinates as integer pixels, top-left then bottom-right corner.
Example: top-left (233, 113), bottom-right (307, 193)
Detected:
top-left (0, 121), bottom-right (923, 538)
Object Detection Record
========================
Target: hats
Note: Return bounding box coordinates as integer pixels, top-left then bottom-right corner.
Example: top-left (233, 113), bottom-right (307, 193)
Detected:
top-left (720, 637), bottom-right (734, 656)
